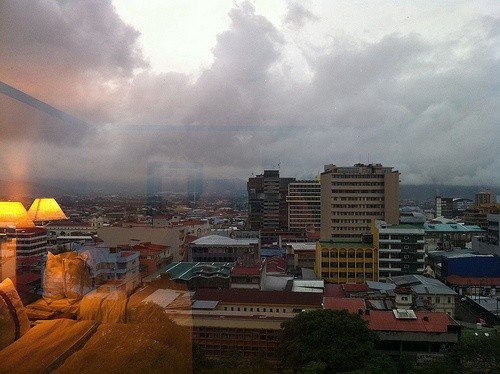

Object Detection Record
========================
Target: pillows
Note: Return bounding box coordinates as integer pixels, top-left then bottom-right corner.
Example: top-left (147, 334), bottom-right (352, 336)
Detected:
top-left (43, 251), bottom-right (70, 304)
top-left (0, 278), bottom-right (30, 352)
top-left (64, 250), bottom-right (96, 303)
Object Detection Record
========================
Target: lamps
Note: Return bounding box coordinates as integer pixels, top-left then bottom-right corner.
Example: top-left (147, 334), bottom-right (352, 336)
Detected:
top-left (0, 201), bottom-right (35, 228)
top-left (27, 198), bottom-right (66, 220)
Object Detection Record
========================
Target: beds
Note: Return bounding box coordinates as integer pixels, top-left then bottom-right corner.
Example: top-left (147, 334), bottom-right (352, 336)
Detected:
top-left (24, 284), bottom-right (190, 323)
top-left (1, 319), bottom-right (193, 374)
